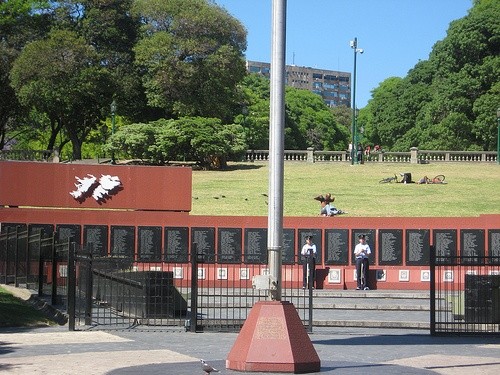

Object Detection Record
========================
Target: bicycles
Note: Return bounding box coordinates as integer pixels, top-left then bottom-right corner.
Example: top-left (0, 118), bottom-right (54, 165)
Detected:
top-left (379, 172), bottom-right (400, 184)
top-left (427, 174), bottom-right (446, 184)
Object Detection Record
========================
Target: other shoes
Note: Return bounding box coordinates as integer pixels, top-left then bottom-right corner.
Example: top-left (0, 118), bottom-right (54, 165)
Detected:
top-left (312, 286), bottom-right (314, 288)
top-left (302, 286), bottom-right (306, 288)
top-left (364, 286), bottom-right (369, 290)
top-left (356, 286), bottom-right (360, 289)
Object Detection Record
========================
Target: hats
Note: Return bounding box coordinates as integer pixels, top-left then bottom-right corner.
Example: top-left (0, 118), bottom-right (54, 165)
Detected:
top-left (305, 235), bottom-right (312, 240)
top-left (358, 234), bottom-right (365, 238)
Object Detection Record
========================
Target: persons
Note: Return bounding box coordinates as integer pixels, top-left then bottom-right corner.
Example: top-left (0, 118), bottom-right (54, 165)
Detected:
top-left (365, 144), bottom-right (370, 153)
top-left (354, 234), bottom-right (371, 290)
top-left (357, 143), bottom-right (362, 161)
top-left (374, 144), bottom-right (379, 151)
top-left (319, 193), bottom-right (335, 216)
top-left (302, 235), bottom-right (317, 289)
top-left (416, 176), bottom-right (429, 184)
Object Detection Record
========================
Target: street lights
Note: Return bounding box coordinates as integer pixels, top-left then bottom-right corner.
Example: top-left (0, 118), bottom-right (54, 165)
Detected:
top-left (350, 37), bottom-right (365, 167)
top-left (111, 100), bottom-right (120, 164)
